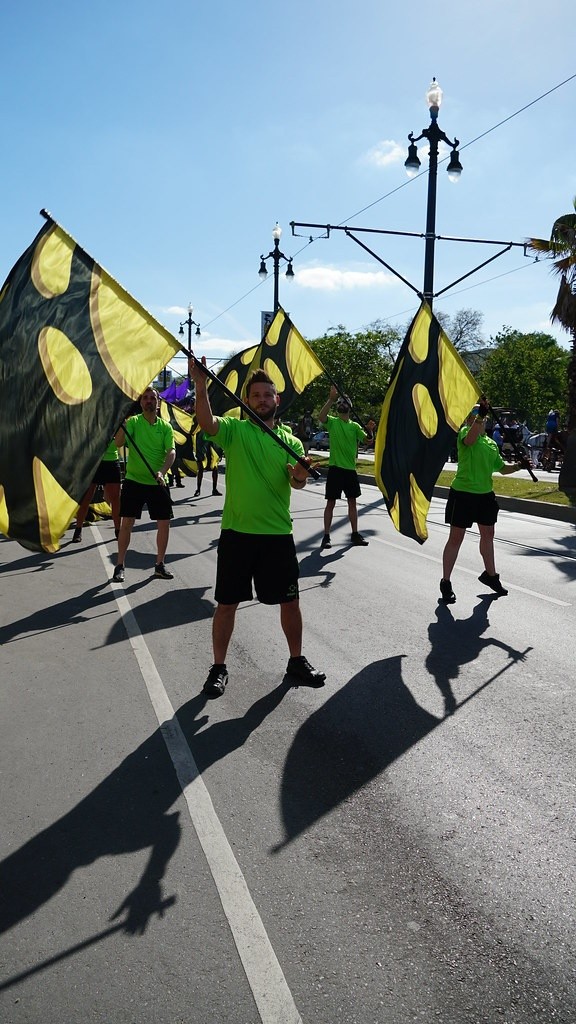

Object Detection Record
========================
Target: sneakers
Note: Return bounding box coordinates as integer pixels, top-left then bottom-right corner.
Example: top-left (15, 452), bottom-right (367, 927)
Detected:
top-left (286, 658), bottom-right (327, 682)
top-left (205, 664), bottom-right (229, 696)
top-left (478, 570), bottom-right (508, 594)
top-left (113, 565), bottom-right (125, 582)
top-left (155, 562), bottom-right (173, 578)
top-left (440, 579), bottom-right (456, 601)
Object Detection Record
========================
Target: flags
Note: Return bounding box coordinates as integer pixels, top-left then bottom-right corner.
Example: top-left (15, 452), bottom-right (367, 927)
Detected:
top-left (374, 299), bottom-right (485, 545)
top-left (1, 219), bottom-right (182, 555)
top-left (240, 308), bottom-right (327, 425)
top-left (159, 399), bottom-right (221, 477)
top-left (74, 493), bottom-right (113, 522)
top-left (207, 344), bottom-right (261, 416)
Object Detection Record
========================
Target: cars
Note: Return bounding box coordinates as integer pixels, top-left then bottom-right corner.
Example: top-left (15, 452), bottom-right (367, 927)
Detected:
top-left (308, 431), bottom-right (330, 451)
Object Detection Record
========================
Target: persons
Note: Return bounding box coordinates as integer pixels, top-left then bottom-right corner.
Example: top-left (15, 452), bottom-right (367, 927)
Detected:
top-left (319, 383), bottom-right (376, 548)
top-left (492, 419), bottom-right (520, 453)
top-left (192, 431), bottom-right (223, 497)
top-left (167, 463), bottom-right (186, 488)
top-left (439, 396), bottom-right (536, 602)
top-left (113, 386), bottom-right (177, 582)
top-left (188, 354), bottom-right (326, 697)
top-left (70, 438), bottom-right (122, 543)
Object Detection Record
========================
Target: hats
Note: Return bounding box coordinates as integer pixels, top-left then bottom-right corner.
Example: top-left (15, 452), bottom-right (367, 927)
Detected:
top-left (495, 424), bottom-right (501, 428)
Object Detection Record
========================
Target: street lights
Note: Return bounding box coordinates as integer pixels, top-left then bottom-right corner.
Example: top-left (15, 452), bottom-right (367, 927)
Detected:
top-left (176, 300), bottom-right (203, 414)
top-left (257, 220), bottom-right (296, 316)
top-left (402, 78), bottom-right (464, 310)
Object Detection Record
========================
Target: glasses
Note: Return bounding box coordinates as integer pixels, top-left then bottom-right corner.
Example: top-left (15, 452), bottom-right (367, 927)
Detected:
top-left (337, 400), bottom-right (347, 405)
top-left (469, 409), bottom-right (479, 415)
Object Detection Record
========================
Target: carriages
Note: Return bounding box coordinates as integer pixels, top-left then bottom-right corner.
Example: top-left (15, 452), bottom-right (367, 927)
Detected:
top-left (512, 419), bottom-right (567, 474)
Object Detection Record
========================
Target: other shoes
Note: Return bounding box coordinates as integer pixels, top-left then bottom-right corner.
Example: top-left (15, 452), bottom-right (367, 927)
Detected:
top-left (72, 531), bottom-right (82, 543)
top-left (213, 488), bottom-right (222, 496)
top-left (321, 538), bottom-right (331, 548)
top-left (350, 533), bottom-right (369, 545)
top-left (115, 531), bottom-right (119, 540)
top-left (194, 490), bottom-right (200, 496)
top-left (176, 483), bottom-right (185, 488)
